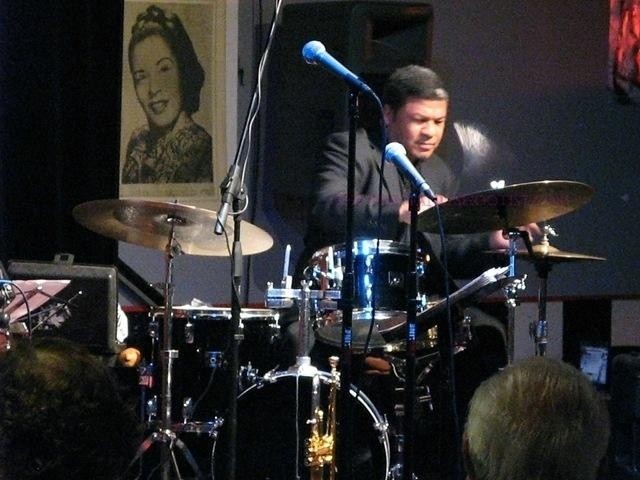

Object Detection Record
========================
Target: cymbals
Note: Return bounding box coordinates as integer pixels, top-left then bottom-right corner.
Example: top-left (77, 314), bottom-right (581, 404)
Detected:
top-left (418, 181), bottom-right (595, 235)
top-left (482, 246), bottom-right (606, 262)
top-left (72, 200), bottom-right (274, 256)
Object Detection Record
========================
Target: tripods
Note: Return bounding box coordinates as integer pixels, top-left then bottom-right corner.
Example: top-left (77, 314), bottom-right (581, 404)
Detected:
top-left (121, 216), bottom-right (205, 479)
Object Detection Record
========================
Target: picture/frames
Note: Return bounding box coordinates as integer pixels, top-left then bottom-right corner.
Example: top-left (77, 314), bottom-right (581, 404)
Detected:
top-left (118, 0), bottom-right (227, 211)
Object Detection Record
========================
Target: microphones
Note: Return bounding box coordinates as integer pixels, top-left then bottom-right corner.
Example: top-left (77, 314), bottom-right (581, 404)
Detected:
top-left (383, 141), bottom-right (437, 203)
top-left (302, 40), bottom-right (377, 99)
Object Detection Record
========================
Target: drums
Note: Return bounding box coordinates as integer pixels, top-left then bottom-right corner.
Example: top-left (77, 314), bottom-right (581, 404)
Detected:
top-left (311, 240), bottom-right (428, 349)
top-left (212, 368), bottom-right (390, 479)
top-left (148, 306), bottom-right (287, 435)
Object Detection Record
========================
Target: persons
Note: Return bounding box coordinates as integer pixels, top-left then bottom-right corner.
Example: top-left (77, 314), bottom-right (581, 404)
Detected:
top-left (278, 65), bottom-right (539, 480)
top-left (121, 5), bottom-right (213, 185)
top-left (460, 354), bottom-right (610, 480)
top-left (0, 335), bottom-right (140, 480)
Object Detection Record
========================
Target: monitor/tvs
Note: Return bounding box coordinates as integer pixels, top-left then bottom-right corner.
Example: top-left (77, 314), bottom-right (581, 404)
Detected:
top-left (574, 341), bottom-right (612, 387)
